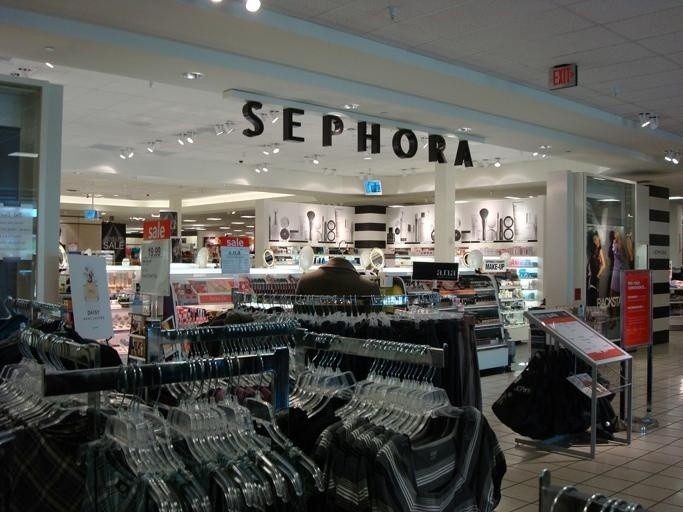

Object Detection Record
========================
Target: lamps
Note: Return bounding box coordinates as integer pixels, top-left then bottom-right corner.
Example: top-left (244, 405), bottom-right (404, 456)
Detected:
top-left (120, 147), bottom-right (134, 159)
top-left (214, 123), bottom-right (233, 136)
top-left (533, 145), bottom-right (551, 158)
top-left (145, 140), bottom-right (162, 152)
top-left (639, 112), bottom-right (659, 130)
top-left (313, 154), bottom-right (319, 164)
top-left (494, 157), bottom-right (501, 167)
top-left (664, 150), bottom-right (680, 164)
top-left (255, 163), bottom-right (268, 173)
top-left (270, 110), bottom-right (279, 123)
top-left (264, 143), bottom-right (279, 155)
top-left (178, 129), bottom-right (198, 145)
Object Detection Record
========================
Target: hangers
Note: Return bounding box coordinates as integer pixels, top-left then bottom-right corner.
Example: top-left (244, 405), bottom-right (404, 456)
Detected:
top-left (0, 278), bottom-right (466, 512)
top-left (550, 485), bottom-right (643, 512)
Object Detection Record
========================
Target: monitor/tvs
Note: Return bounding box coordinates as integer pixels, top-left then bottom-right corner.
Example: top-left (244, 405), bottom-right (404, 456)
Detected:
top-left (364, 180), bottom-right (382, 195)
top-left (84, 210), bottom-right (99, 220)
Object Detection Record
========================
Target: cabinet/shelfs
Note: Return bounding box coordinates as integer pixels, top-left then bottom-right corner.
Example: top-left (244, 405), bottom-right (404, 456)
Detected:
top-left (234, 241), bottom-right (366, 279)
top-left (58, 242), bottom-right (234, 355)
top-left (378, 243), bottom-right (541, 377)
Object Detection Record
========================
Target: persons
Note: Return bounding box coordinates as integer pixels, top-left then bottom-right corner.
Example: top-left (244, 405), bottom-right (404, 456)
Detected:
top-left (196, 244), bottom-right (212, 264)
top-left (610, 230), bottom-right (630, 317)
top-left (585, 231), bottom-right (606, 306)
